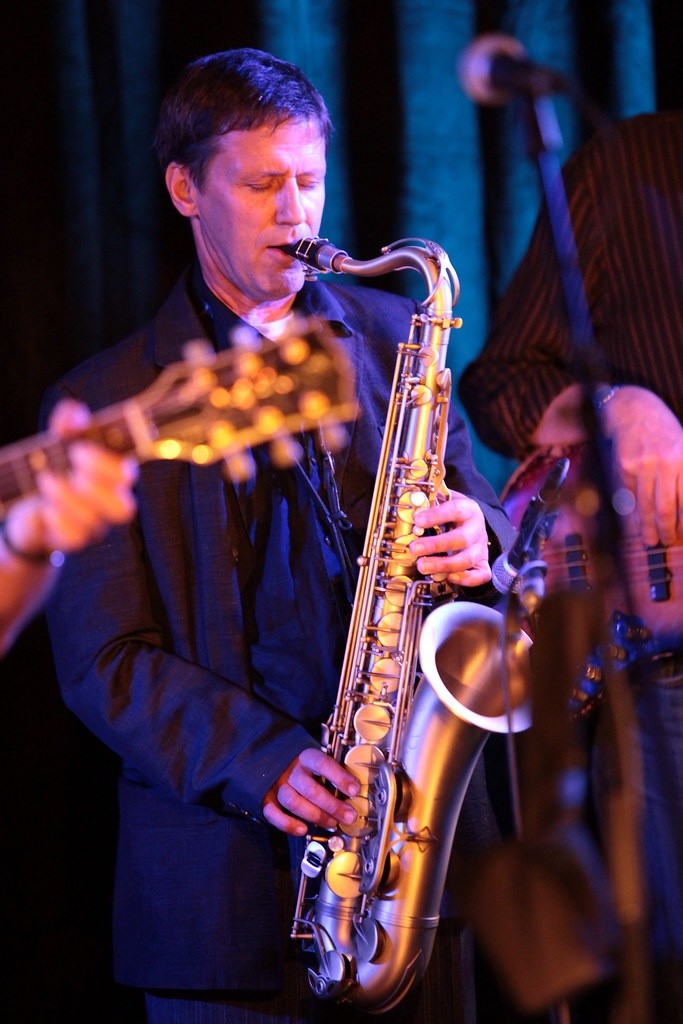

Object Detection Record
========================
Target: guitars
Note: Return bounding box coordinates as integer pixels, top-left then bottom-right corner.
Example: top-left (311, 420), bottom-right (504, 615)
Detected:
top-left (497, 437), bottom-right (683, 723)
top-left (0, 312), bottom-right (363, 521)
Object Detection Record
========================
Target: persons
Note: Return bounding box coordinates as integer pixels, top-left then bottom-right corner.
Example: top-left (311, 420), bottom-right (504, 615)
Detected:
top-left (38, 46), bottom-right (521, 1024)
top-left (0, 399), bottom-right (136, 656)
top-left (456, 78), bottom-right (683, 993)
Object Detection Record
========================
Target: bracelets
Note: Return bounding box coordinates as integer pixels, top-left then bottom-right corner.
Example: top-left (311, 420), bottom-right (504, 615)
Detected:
top-left (598, 385), bottom-right (619, 409)
top-left (0, 518), bottom-right (67, 568)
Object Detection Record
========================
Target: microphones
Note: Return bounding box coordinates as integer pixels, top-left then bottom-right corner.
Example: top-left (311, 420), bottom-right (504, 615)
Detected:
top-left (459, 33), bottom-right (564, 104)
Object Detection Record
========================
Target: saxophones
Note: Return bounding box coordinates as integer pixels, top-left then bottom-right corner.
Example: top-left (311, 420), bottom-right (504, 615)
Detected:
top-left (273, 235), bottom-right (543, 1017)
